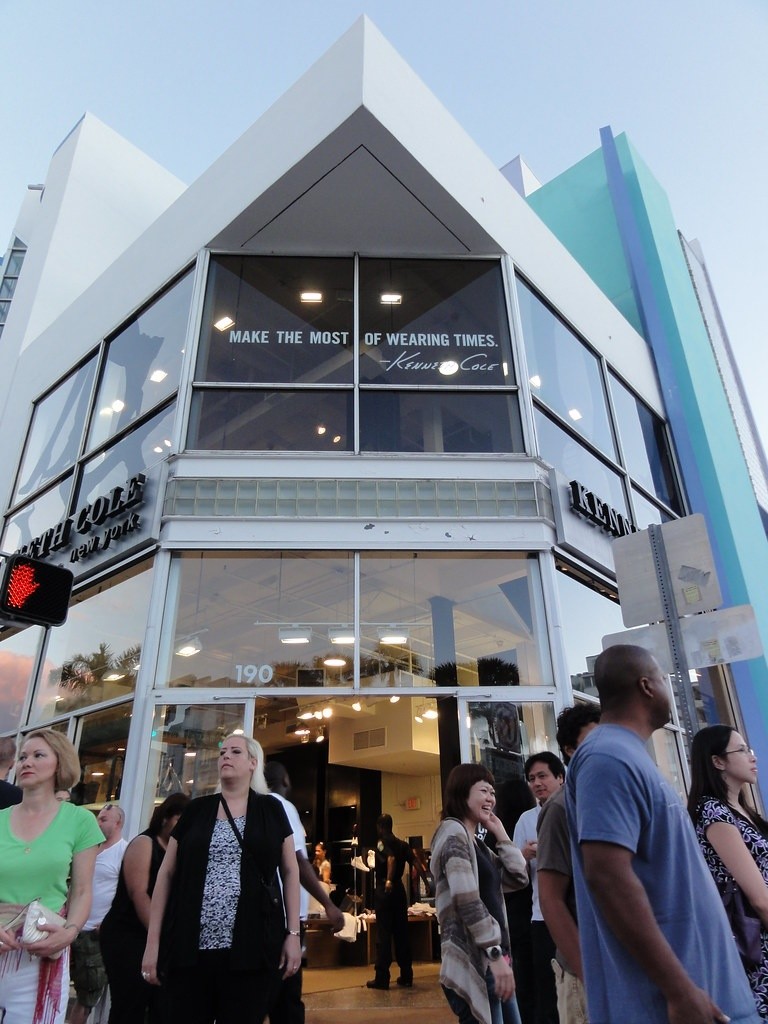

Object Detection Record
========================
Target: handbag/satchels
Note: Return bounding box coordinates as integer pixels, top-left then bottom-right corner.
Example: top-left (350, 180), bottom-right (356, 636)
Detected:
top-left (4, 894), bottom-right (66, 960)
top-left (693, 799), bottom-right (763, 966)
top-left (262, 886), bottom-right (287, 943)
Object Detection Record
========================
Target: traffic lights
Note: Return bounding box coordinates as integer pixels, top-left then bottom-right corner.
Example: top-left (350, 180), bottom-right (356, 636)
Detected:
top-left (0, 552), bottom-right (75, 628)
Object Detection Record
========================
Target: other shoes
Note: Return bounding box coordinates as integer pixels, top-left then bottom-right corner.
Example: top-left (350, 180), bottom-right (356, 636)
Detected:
top-left (367, 977), bottom-right (389, 989)
top-left (397, 974), bottom-right (413, 987)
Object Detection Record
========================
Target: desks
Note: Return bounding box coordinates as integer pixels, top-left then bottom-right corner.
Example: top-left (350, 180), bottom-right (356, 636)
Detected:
top-left (302, 913), bottom-right (441, 971)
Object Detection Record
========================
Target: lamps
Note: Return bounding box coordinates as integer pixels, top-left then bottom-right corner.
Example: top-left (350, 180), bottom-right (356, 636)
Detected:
top-left (184, 744), bottom-right (197, 757)
top-left (91, 769), bottom-right (104, 775)
top-left (327, 625), bottom-right (362, 645)
top-left (317, 425), bottom-right (326, 436)
top-left (298, 289), bottom-right (324, 304)
top-left (278, 625), bottom-right (312, 645)
top-left (294, 720), bottom-right (311, 735)
top-left (335, 288), bottom-right (353, 303)
top-left (331, 433), bottom-right (341, 443)
top-left (101, 663), bottom-right (128, 682)
top-left (414, 708), bottom-right (424, 724)
top-left (301, 734), bottom-right (309, 743)
top-left (438, 357), bottom-right (459, 375)
top-left (174, 636), bottom-right (202, 658)
top-left (314, 731), bottom-right (325, 743)
top-left (377, 625), bottom-right (410, 646)
top-left (314, 695), bottom-right (400, 720)
top-left (377, 289), bottom-right (404, 305)
top-left (422, 704), bottom-right (438, 720)
top-left (296, 704), bottom-right (316, 719)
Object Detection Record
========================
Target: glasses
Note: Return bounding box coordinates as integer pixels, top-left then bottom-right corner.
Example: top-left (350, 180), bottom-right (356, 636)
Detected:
top-left (102, 804), bottom-right (112, 811)
top-left (721, 747), bottom-right (754, 756)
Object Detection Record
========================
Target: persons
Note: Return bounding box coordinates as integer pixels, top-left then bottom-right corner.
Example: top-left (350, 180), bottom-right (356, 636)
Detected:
top-left (512, 752), bottom-right (566, 1024)
top-left (564, 644), bottom-right (762, 1024)
top-left (688, 725), bottom-right (768, 1024)
top-left (263, 762), bottom-right (345, 1024)
top-left (429, 764), bottom-right (529, 1024)
top-left (313, 842), bottom-right (332, 885)
top-left (535, 703), bottom-right (602, 1024)
top-left (142, 734), bottom-right (302, 1024)
top-left (366, 814), bottom-right (430, 989)
top-left (0, 729), bottom-right (191, 1024)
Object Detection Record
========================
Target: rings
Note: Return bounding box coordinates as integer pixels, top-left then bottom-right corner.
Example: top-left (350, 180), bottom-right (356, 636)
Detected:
top-left (143, 972), bottom-right (147, 979)
top-left (294, 967), bottom-right (297, 970)
top-left (0, 941), bottom-right (3, 946)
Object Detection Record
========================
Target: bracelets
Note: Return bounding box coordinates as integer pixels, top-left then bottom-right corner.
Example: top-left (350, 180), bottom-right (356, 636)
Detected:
top-left (386, 880), bottom-right (391, 884)
top-left (287, 931), bottom-right (300, 935)
top-left (65, 924), bottom-right (79, 933)
